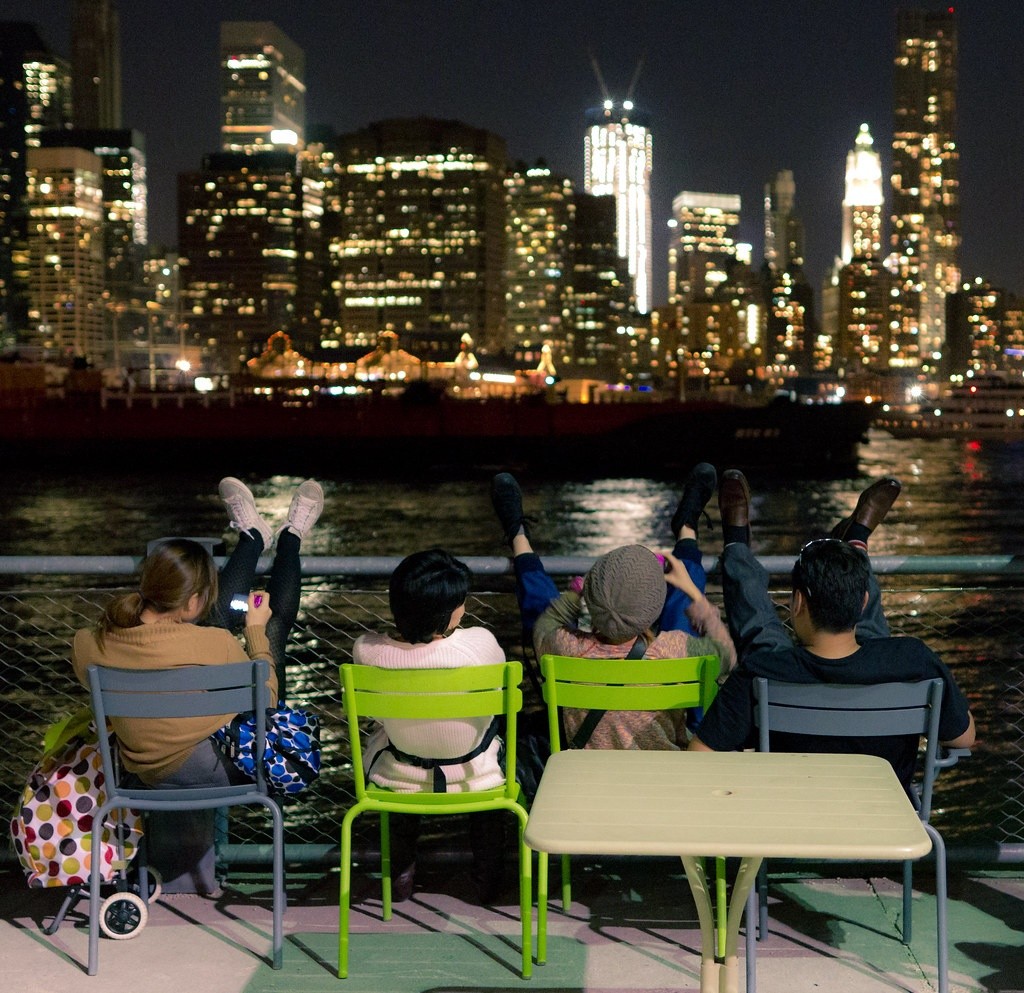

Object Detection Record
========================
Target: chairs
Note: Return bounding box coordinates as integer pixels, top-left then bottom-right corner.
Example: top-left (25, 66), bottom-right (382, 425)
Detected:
top-left (85, 657), bottom-right (288, 971)
top-left (543, 651), bottom-right (728, 960)
top-left (747, 670), bottom-right (950, 990)
top-left (334, 660), bottom-right (547, 975)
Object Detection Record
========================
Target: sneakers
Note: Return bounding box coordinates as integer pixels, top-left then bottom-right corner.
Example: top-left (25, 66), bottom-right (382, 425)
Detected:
top-left (490, 472), bottom-right (531, 546)
top-left (217, 477), bottom-right (274, 553)
top-left (274, 480), bottom-right (325, 552)
top-left (671, 462), bottom-right (717, 539)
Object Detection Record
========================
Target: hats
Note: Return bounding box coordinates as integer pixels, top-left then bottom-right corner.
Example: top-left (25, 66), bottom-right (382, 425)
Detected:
top-left (582, 545), bottom-right (667, 640)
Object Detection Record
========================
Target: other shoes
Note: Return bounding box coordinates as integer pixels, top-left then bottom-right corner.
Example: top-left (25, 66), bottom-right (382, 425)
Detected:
top-left (473, 864), bottom-right (508, 905)
top-left (392, 861), bottom-right (416, 902)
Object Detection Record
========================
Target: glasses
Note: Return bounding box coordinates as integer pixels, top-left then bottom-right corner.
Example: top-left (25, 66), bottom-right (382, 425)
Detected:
top-left (800, 539), bottom-right (843, 597)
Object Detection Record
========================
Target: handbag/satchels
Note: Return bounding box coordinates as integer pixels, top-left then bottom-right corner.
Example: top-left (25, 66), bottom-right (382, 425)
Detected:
top-left (211, 702), bottom-right (322, 795)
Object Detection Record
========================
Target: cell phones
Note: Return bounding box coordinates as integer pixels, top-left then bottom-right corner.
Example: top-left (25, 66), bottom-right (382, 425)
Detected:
top-left (230, 593), bottom-right (262, 613)
top-left (655, 554), bottom-right (671, 573)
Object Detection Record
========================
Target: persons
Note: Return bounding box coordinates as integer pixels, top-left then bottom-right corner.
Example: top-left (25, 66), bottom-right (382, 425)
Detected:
top-left (352, 549), bottom-right (508, 904)
top-left (683, 470), bottom-right (975, 793)
top-left (71, 477), bottom-right (325, 789)
top-left (488, 461), bottom-right (737, 753)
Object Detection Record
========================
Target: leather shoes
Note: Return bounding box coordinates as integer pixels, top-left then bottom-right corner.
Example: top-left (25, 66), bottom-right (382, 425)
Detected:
top-left (832, 479), bottom-right (902, 542)
top-left (718, 469), bottom-right (752, 549)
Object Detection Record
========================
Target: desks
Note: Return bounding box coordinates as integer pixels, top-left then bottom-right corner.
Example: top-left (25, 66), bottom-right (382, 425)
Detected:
top-left (529, 744), bottom-right (937, 993)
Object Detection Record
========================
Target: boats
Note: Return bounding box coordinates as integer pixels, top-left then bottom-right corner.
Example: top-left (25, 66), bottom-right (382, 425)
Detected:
top-left (0, 347), bottom-right (878, 475)
top-left (880, 370), bottom-right (1024, 442)
